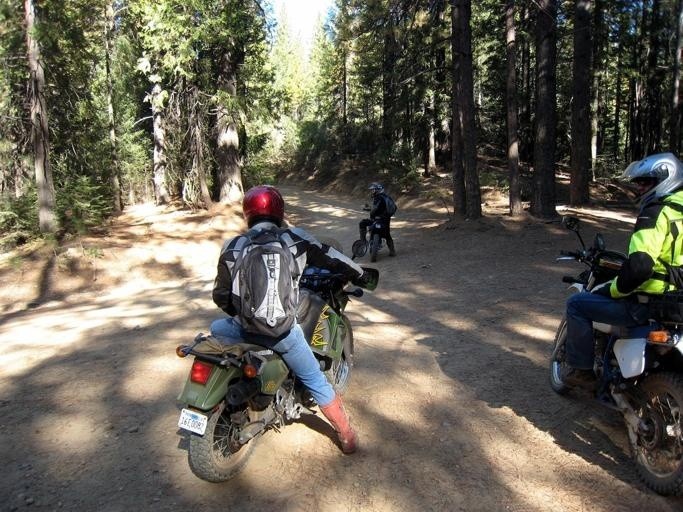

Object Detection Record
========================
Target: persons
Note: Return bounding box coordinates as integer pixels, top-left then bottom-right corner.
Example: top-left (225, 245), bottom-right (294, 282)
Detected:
top-left (553, 149), bottom-right (683, 399)
top-left (206, 185), bottom-right (371, 456)
top-left (356, 181), bottom-right (396, 257)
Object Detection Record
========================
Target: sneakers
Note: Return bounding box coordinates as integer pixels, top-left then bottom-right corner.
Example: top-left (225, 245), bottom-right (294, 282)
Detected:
top-left (561, 367), bottom-right (596, 387)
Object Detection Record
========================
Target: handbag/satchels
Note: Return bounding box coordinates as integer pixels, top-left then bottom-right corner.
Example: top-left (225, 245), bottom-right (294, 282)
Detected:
top-left (381, 194), bottom-right (396, 217)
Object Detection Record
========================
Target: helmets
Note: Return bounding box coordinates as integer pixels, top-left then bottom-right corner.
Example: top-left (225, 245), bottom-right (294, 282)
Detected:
top-left (624, 152), bottom-right (683, 208)
top-left (242, 185), bottom-right (285, 227)
top-left (369, 182), bottom-right (385, 198)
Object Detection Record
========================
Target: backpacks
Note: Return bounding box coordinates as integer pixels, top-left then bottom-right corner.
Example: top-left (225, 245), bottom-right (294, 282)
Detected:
top-left (239, 226), bottom-right (301, 338)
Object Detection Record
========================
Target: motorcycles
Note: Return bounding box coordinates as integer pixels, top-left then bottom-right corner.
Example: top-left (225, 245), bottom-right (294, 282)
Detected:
top-left (350, 203), bottom-right (385, 263)
top-left (175, 253), bottom-right (380, 486)
top-left (549, 214), bottom-right (681, 498)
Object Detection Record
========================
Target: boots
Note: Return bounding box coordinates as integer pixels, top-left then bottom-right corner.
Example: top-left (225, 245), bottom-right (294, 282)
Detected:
top-left (320, 395), bottom-right (357, 453)
top-left (387, 239), bottom-right (395, 256)
top-left (359, 228), bottom-right (366, 240)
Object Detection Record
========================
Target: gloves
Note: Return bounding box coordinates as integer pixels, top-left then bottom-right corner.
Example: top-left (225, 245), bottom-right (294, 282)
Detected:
top-left (590, 279), bottom-right (613, 298)
